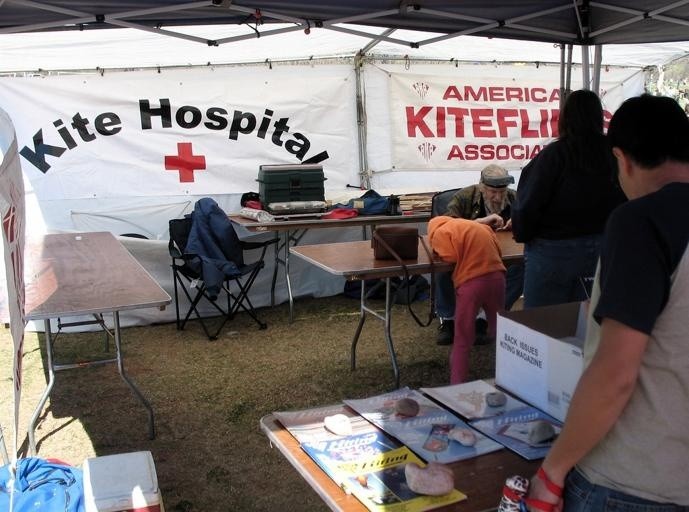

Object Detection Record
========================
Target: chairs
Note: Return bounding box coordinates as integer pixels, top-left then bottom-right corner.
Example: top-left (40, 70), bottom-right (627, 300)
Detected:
top-left (168, 218), bottom-right (281, 342)
top-left (430, 188), bottom-right (462, 222)
top-left (580, 271), bottom-right (595, 300)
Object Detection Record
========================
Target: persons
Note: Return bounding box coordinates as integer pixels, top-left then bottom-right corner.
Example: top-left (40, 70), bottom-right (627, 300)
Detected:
top-left (427, 214), bottom-right (510, 386)
top-left (512, 88), bottom-right (627, 310)
top-left (522, 93), bottom-right (689, 512)
top-left (435, 162), bottom-right (525, 345)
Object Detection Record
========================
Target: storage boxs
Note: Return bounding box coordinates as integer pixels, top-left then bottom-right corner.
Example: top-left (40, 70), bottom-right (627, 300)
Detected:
top-left (83, 451), bottom-right (165, 511)
top-left (495, 300), bottom-right (589, 425)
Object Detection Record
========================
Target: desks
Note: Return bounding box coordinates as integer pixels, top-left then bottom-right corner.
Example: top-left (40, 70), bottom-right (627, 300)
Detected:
top-left (288, 228), bottom-right (525, 391)
top-left (226, 192), bottom-right (441, 323)
top-left (258, 378), bottom-right (567, 512)
top-left (23, 231), bottom-right (173, 457)
